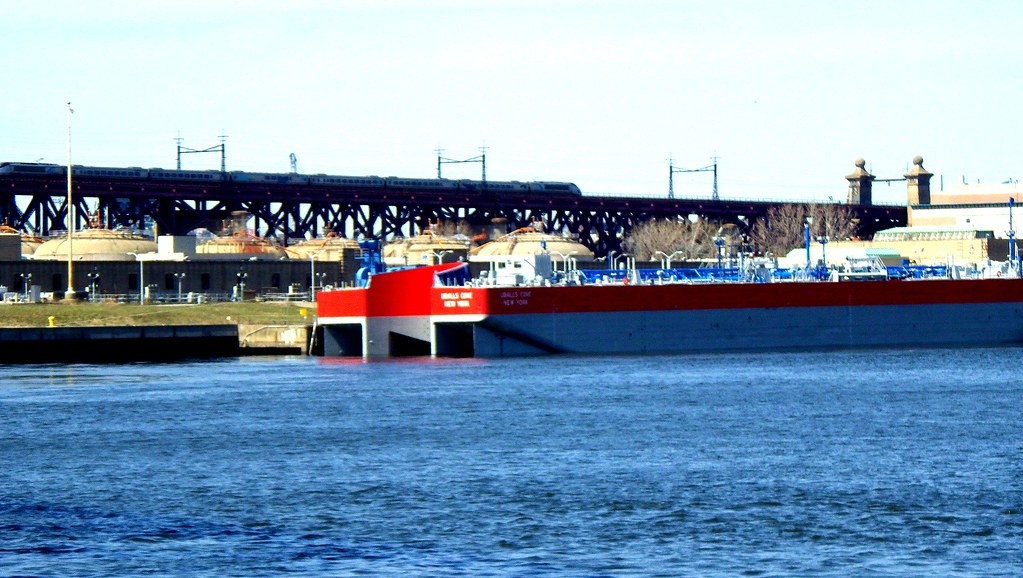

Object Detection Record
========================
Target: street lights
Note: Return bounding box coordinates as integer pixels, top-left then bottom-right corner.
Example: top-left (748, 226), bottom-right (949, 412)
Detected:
top-left (20, 273), bottom-right (33, 301)
top-left (126, 251), bottom-right (144, 307)
top-left (316, 272), bottom-right (326, 288)
top-left (174, 273), bottom-right (186, 304)
top-left (237, 272), bottom-right (248, 301)
top-left (88, 273), bottom-right (100, 304)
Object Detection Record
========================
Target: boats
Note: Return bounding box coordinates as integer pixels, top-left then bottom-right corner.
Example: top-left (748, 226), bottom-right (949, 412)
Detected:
top-left (316, 194), bottom-right (1023, 358)
